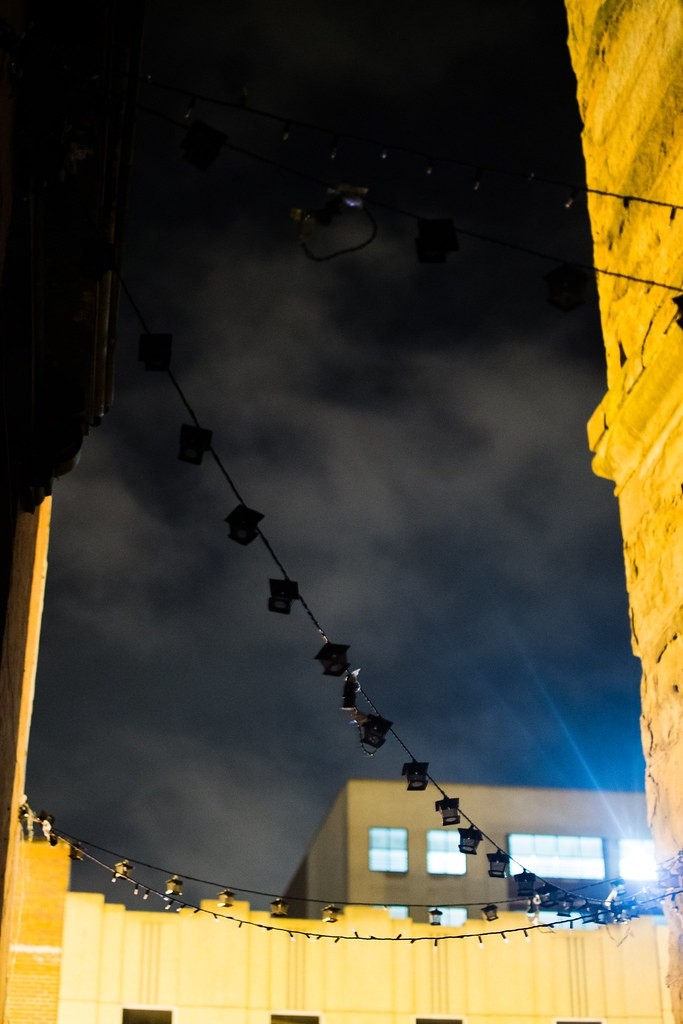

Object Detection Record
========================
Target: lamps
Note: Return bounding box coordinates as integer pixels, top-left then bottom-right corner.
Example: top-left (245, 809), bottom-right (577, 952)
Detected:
top-left (181, 100), bottom-right (593, 312)
top-left (486, 849), bottom-right (510, 878)
top-left (399, 764), bottom-right (430, 791)
top-left (173, 423), bottom-right (214, 468)
top-left (267, 579), bottom-right (300, 616)
top-left (70, 842), bottom-right (289, 917)
top-left (356, 715), bottom-right (394, 748)
top-left (515, 871), bottom-right (606, 926)
top-left (321, 903), bottom-right (341, 923)
top-left (482, 905), bottom-right (500, 921)
top-left (437, 798), bottom-right (461, 826)
top-left (135, 332), bottom-right (176, 374)
top-left (341, 678), bottom-right (357, 712)
top-left (456, 827), bottom-right (481, 853)
top-left (312, 642), bottom-right (350, 678)
top-left (429, 907), bottom-right (442, 924)
top-left (223, 505), bottom-right (267, 546)
